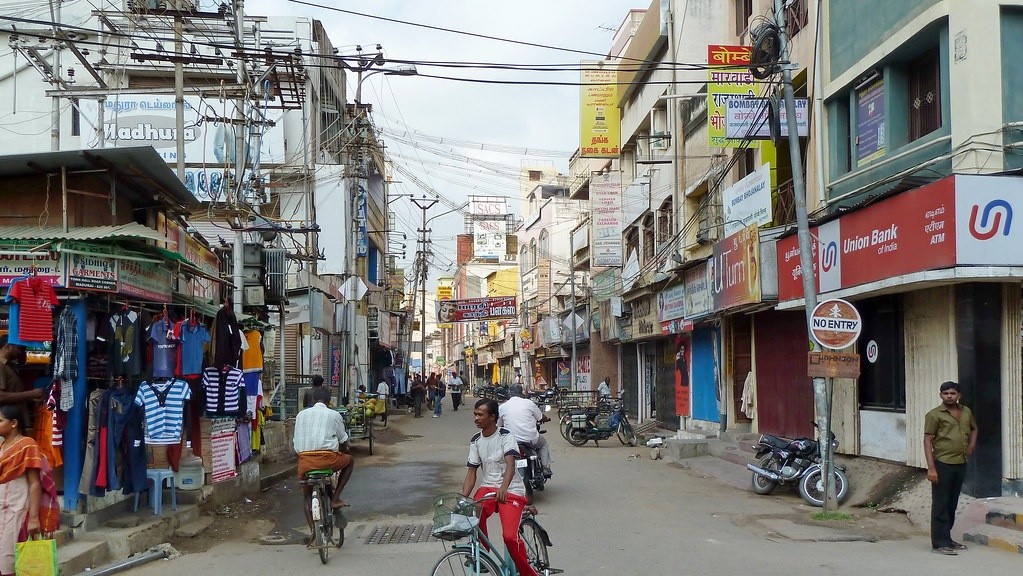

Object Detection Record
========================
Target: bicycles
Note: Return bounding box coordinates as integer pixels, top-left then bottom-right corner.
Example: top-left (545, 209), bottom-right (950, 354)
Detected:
top-left (559, 391), bottom-right (624, 442)
top-left (299, 469), bottom-right (348, 564)
top-left (477, 382), bottom-right (509, 402)
top-left (430, 492), bottom-right (564, 576)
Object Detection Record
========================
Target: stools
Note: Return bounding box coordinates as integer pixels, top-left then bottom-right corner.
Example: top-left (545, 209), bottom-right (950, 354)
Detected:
top-left (135, 469), bottom-right (175, 514)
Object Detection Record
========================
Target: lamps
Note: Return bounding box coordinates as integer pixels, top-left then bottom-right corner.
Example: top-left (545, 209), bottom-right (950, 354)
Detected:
top-left (367, 229), bottom-right (407, 259)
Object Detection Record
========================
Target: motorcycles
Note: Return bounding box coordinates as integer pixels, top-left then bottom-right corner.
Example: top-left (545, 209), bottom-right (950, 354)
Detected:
top-left (527, 385), bottom-right (566, 405)
top-left (746, 420), bottom-right (849, 507)
top-left (515, 405), bottom-right (551, 505)
top-left (564, 389), bottom-right (637, 447)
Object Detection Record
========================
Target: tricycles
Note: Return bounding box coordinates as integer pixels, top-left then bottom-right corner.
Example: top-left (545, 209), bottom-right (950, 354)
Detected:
top-left (332, 406), bottom-right (375, 455)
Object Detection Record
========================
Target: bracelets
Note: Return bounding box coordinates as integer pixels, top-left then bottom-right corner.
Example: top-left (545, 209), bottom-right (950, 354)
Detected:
top-left (435, 386), bottom-right (437, 389)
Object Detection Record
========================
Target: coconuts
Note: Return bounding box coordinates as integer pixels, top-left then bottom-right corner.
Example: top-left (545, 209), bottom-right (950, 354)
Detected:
top-left (340, 398), bottom-right (378, 425)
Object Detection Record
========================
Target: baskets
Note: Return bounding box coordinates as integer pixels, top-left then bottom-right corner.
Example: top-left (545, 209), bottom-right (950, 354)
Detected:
top-left (427, 491), bottom-right (483, 541)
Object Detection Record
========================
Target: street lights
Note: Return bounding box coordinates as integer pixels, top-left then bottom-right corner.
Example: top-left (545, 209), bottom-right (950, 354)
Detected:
top-left (351, 64), bottom-right (419, 366)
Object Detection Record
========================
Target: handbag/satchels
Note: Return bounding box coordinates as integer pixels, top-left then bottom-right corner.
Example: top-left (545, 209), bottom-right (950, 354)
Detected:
top-left (14, 532), bottom-right (59, 576)
top-left (440, 384), bottom-right (446, 397)
top-left (452, 386), bottom-right (459, 391)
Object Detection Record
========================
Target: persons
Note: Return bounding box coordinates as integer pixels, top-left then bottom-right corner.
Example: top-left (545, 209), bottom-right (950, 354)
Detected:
top-left (293, 385), bottom-right (354, 532)
top-left (447, 372), bottom-right (463, 411)
top-left (459, 369), bottom-right (468, 405)
top-left (0, 407), bottom-right (43, 576)
top-left (514, 374), bottom-right (522, 382)
top-left (358, 385), bottom-right (366, 393)
top-left (455, 397), bottom-right (539, 576)
top-left (495, 382), bottom-right (553, 479)
top-left (302, 375), bottom-right (324, 408)
top-left (375, 375), bottom-right (390, 421)
top-left (438, 301), bottom-right (456, 323)
top-left (0, 333), bottom-right (45, 435)
top-left (598, 376), bottom-right (612, 399)
top-left (924, 381), bottom-right (978, 555)
top-left (408, 372), bottom-right (443, 418)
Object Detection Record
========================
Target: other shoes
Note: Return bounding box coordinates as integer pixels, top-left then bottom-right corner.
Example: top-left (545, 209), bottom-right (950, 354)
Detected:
top-left (433, 415), bottom-right (441, 418)
top-left (949, 539), bottom-right (966, 550)
top-left (415, 414), bottom-right (423, 418)
top-left (543, 468), bottom-right (553, 476)
top-left (932, 546), bottom-right (957, 554)
top-left (464, 560), bottom-right (489, 573)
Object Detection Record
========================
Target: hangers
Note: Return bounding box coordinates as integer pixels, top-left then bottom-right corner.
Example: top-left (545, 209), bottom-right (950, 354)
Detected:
top-left (158, 300), bottom-right (250, 333)
top-left (65, 293), bottom-right (71, 306)
top-left (28, 267), bottom-right (38, 279)
top-left (152, 376), bottom-right (169, 381)
top-left (221, 362), bottom-right (230, 371)
top-left (91, 376), bottom-right (123, 390)
top-left (118, 298), bottom-right (130, 313)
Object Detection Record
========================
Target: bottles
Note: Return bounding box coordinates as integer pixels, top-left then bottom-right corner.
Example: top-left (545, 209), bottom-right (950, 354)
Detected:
top-left (178, 447), bottom-right (203, 491)
